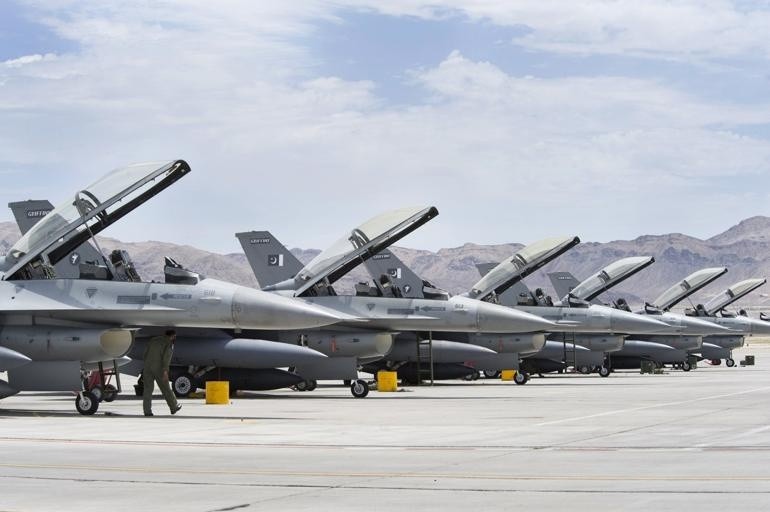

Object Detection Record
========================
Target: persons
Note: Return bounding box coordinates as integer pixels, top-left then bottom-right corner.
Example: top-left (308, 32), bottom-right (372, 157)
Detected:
top-left (142, 329), bottom-right (182, 416)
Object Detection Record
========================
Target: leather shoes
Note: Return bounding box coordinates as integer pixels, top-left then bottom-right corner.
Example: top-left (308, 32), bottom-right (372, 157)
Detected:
top-left (171, 403), bottom-right (182, 414)
top-left (145, 413), bottom-right (153, 416)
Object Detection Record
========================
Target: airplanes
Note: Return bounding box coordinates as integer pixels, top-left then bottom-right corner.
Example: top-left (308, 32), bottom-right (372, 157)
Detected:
top-left (0, 159), bottom-right (770, 415)
